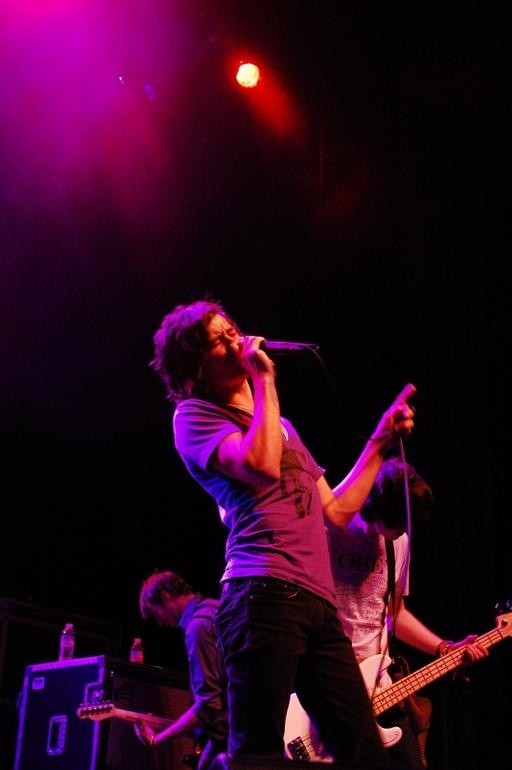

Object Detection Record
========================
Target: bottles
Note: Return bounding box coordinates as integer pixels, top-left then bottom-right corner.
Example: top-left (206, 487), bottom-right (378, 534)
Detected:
top-left (130, 637), bottom-right (144, 663)
top-left (59, 623), bottom-right (76, 660)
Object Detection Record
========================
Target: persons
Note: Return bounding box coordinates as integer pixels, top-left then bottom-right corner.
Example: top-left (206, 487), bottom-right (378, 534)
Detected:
top-left (148, 299), bottom-right (418, 770)
top-left (132, 570), bottom-right (230, 770)
top-left (309, 456), bottom-right (491, 770)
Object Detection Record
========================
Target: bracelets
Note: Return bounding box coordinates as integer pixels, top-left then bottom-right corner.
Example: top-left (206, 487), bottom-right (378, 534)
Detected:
top-left (433, 638), bottom-right (454, 660)
top-left (149, 734), bottom-right (155, 747)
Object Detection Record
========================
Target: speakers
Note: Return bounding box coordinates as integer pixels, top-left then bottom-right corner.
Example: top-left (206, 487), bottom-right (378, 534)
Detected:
top-left (15, 651), bottom-right (197, 769)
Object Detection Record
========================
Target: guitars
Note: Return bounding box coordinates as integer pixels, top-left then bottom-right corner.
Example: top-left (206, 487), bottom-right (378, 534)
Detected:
top-left (283, 603), bottom-right (512, 762)
top-left (77, 701), bottom-right (203, 767)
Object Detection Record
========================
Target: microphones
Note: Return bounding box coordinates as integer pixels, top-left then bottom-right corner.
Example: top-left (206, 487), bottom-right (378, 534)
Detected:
top-left (260, 340), bottom-right (320, 359)
